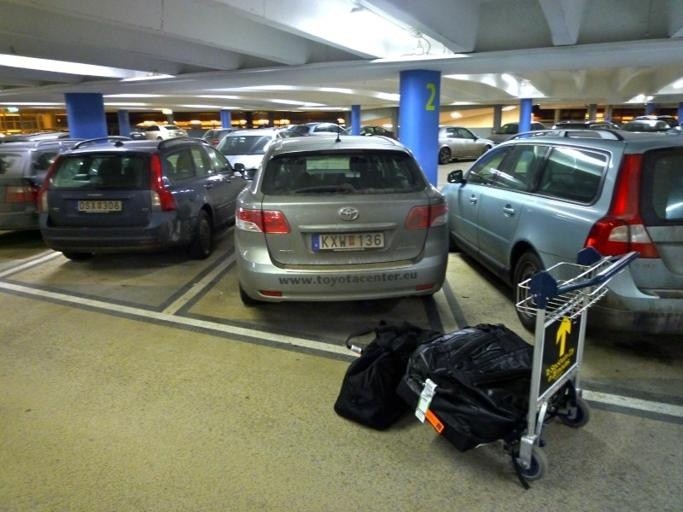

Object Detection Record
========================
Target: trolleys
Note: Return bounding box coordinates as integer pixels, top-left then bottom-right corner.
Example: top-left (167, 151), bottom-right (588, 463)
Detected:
top-left (389, 247), bottom-right (639, 481)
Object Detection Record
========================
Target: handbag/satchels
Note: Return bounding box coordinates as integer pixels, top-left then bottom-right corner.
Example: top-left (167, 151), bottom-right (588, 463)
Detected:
top-left (332, 318), bottom-right (445, 432)
top-left (396, 319), bottom-right (534, 443)
